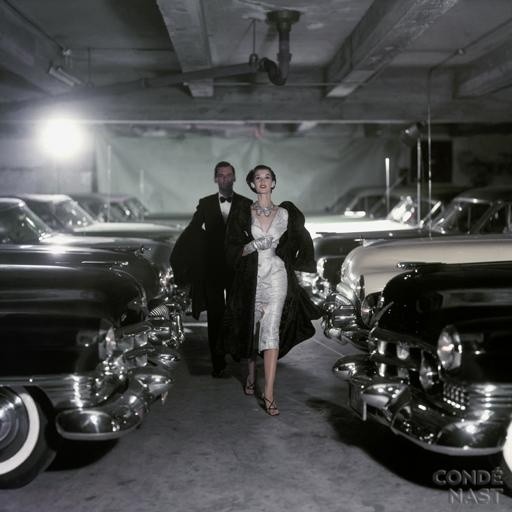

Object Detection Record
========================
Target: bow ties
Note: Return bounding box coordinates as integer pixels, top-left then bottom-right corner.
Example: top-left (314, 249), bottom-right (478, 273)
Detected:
top-left (220, 195), bottom-right (232, 204)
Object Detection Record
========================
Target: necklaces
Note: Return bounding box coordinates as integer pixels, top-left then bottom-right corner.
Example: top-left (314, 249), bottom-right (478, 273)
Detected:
top-left (252, 202), bottom-right (274, 217)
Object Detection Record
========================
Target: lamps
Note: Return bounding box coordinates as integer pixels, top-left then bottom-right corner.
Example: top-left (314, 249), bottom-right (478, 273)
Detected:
top-left (398, 122), bottom-right (425, 224)
top-left (48, 56), bottom-right (84, 88)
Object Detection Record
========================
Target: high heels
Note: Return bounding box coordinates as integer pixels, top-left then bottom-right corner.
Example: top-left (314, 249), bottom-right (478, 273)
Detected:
top-left (244, 377), bottom-right (257, 396)
top-left (260, 393), bottom-right (280, 417)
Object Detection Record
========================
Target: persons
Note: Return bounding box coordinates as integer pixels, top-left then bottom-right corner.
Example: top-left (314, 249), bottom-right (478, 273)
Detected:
top-left (170, 161), bottom-right (253, 377)
top-left (225, 165), bottom-right (329, 415)
